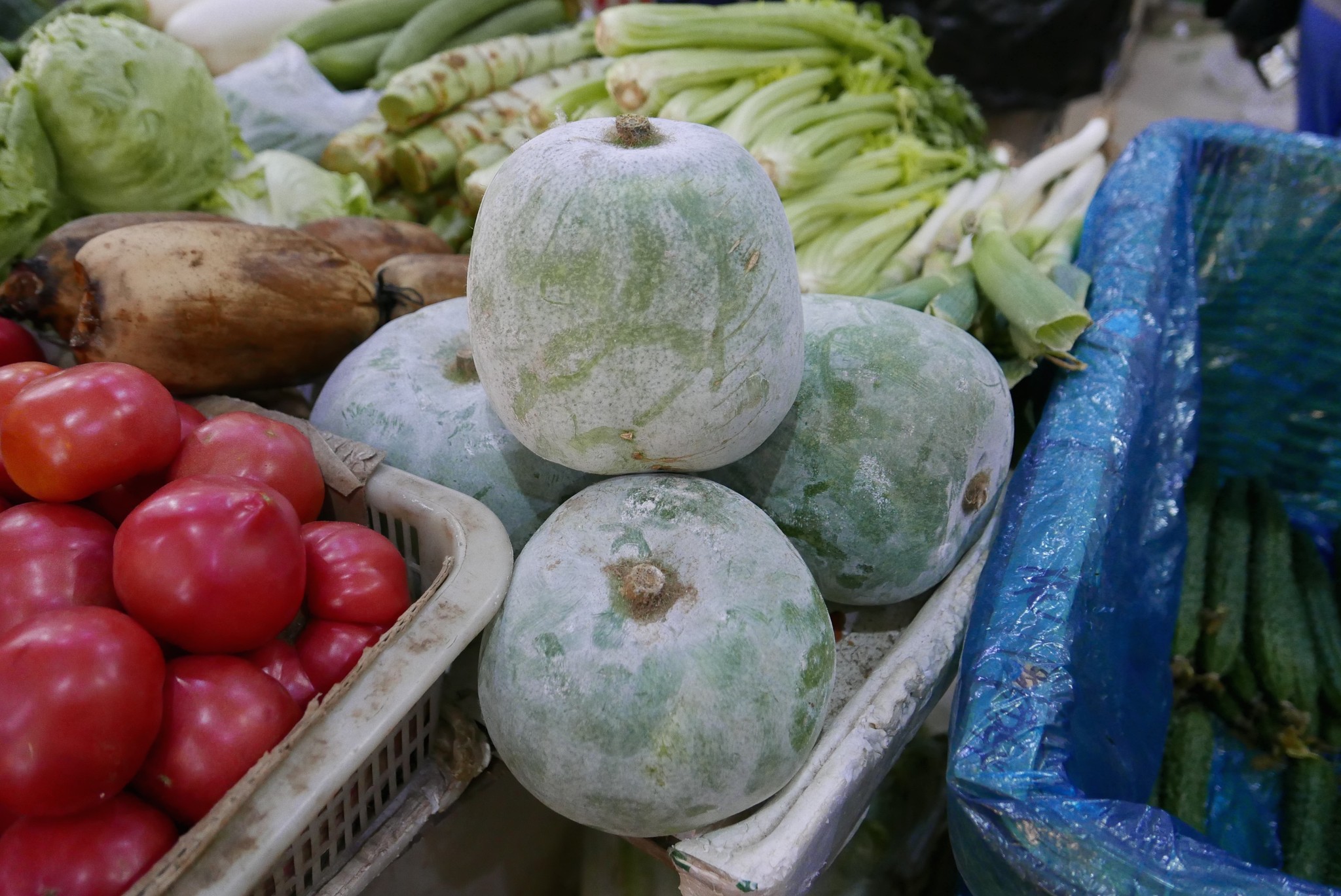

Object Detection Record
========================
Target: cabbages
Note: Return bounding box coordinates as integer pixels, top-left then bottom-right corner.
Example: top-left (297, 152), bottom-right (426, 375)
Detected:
top-left (0, 10), bottom-right (374, 278)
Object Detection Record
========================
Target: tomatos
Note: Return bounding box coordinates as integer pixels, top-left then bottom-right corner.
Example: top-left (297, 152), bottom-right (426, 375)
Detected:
top-left (0, 318), bottom-right (409, 895)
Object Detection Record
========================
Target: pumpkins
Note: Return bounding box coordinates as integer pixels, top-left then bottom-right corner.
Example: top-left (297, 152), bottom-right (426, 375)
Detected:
top-left (306, 111), bottom-right (1019, 844)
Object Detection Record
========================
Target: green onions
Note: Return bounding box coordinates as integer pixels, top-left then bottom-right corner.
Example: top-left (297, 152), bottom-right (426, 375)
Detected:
top-left (871, 117), bottom-right (1109, 388)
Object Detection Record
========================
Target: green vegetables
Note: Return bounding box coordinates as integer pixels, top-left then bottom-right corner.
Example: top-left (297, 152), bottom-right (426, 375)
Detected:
top-left (595, 5), bottom-right (986, 295)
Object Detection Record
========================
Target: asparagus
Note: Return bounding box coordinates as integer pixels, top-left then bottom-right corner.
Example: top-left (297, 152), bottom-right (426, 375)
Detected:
top-left (317, 25), bottom-right (611, 256)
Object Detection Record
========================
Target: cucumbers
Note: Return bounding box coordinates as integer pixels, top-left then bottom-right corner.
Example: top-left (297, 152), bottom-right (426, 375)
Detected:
top-left (1151, 477), bottom-right (1340, 890)
